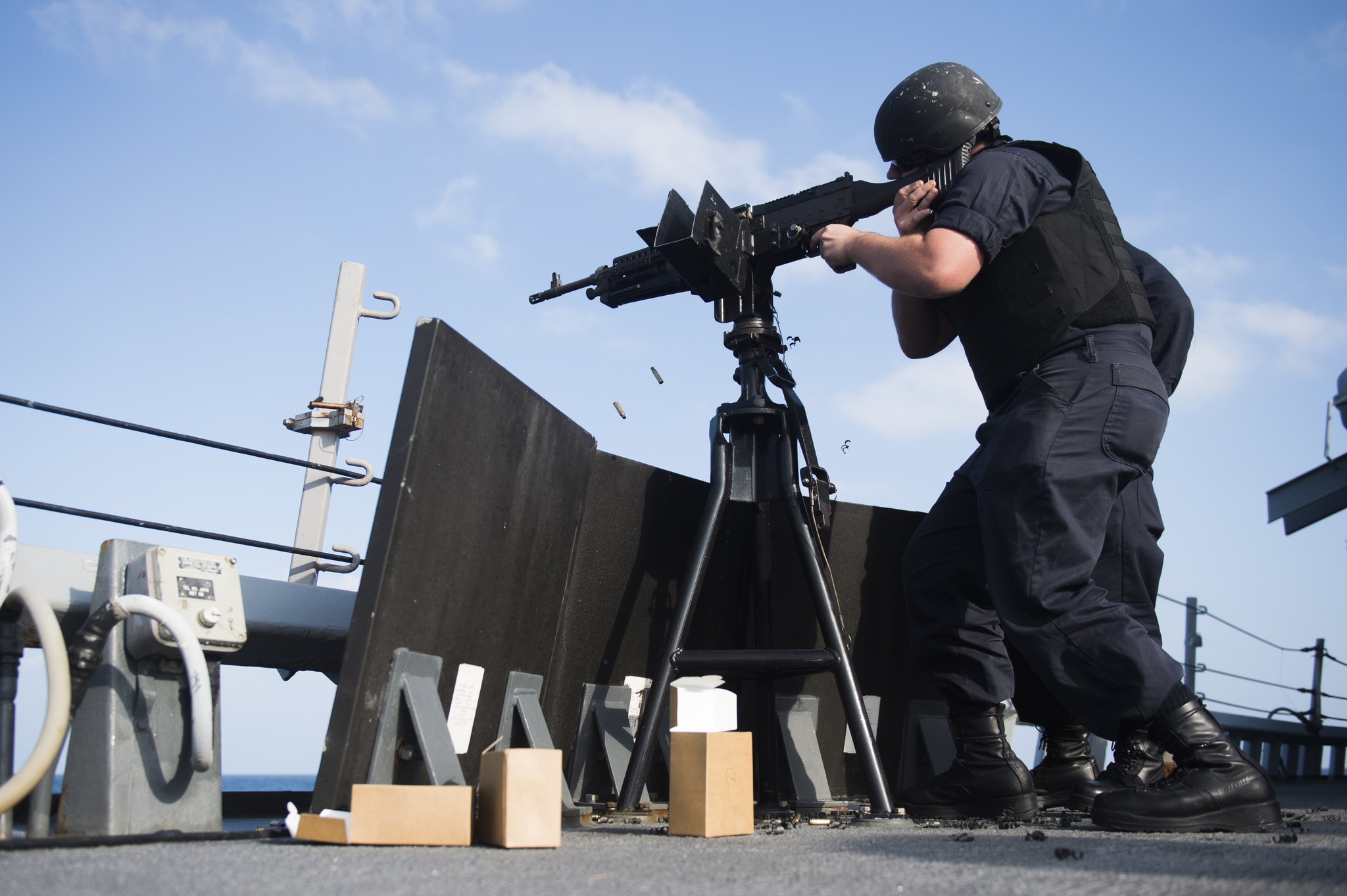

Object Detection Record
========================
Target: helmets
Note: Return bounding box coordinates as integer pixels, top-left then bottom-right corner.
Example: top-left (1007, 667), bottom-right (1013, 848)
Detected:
top-left (874, 63), bottom-right (1003, 162)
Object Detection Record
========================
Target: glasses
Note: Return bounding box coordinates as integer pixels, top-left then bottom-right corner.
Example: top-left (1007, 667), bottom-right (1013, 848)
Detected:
top-left (890, 150), bottom-right (934, 173)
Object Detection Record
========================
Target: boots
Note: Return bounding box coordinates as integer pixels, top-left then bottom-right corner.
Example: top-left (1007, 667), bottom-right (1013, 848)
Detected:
top-left (1028, 724), bottom-right (1099, 810)
top-left (1069, 730), bottom-right (1167, 808)
top-left (891, 703), bottom-right (1037, 824)
top-left (1091, 693), bottom-right (1284, 832)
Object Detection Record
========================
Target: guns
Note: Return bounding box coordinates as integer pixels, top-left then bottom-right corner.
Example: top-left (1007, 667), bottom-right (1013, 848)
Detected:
top-left (526, 137), bottom-right (971, 313)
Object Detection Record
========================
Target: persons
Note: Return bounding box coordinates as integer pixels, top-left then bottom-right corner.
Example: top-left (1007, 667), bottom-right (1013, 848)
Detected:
top-left (806, 60), bottom-right (1285, 833)
top-left (1027, 239), bottom-right (1195, 811)
